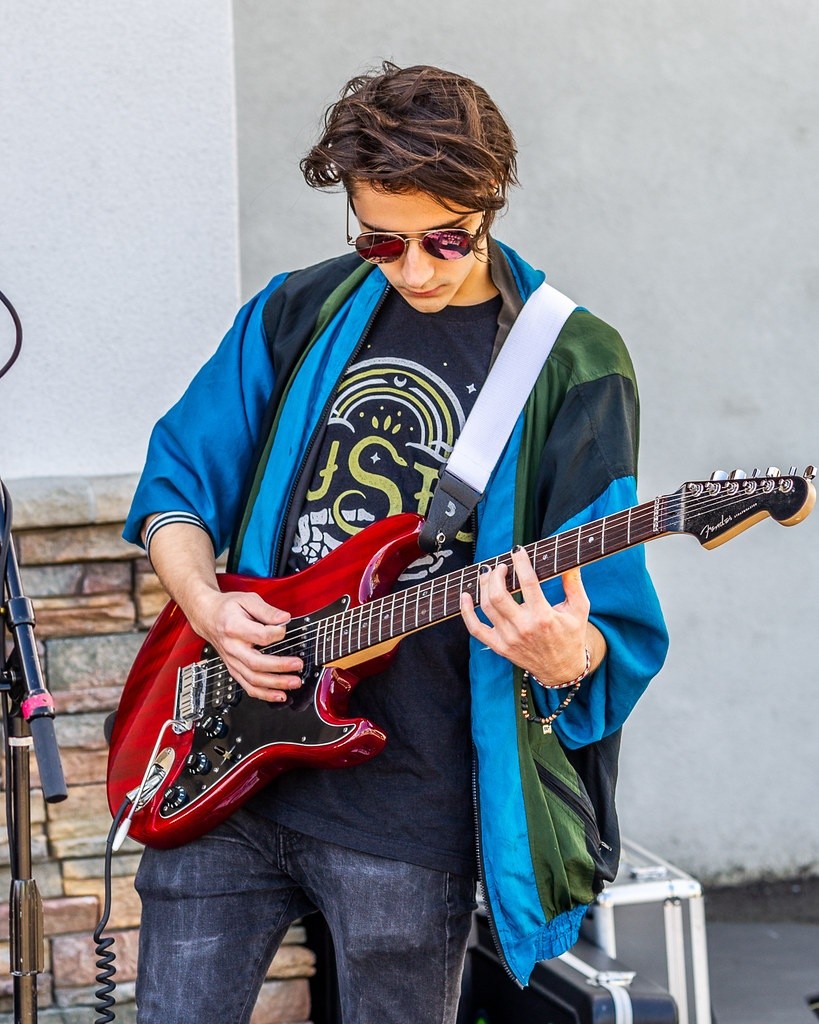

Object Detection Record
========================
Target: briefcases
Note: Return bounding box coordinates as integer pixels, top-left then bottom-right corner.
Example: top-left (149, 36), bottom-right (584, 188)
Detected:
top-left (456, 909), bottom-right (679, 1024)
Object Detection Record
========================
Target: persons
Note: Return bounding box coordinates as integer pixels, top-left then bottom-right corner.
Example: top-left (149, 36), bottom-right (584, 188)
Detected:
top-left (122, 64), bottom-right (668, 1024)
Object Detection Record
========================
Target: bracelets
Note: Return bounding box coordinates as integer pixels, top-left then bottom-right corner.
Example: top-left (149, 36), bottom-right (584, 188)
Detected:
top-left (520, 671), bottom-right (580, 735)
top-left (145, 510), bottom-right (215, 575)
top-left (532, 647), bottom-right (590, 689)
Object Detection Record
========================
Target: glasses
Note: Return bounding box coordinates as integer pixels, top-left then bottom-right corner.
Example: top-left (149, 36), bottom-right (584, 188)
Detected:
top-left (346, 192), bottom-right (486, 265)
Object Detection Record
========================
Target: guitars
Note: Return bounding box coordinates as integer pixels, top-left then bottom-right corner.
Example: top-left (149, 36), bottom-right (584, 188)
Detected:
top-left (105, 464), bottom-right (818, 851)
